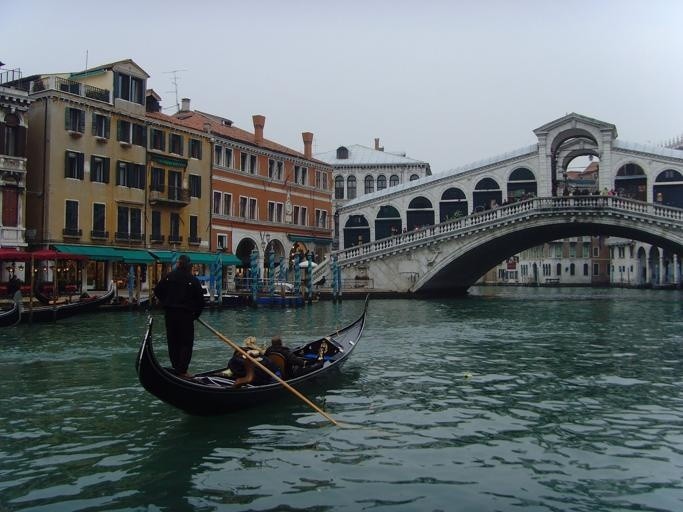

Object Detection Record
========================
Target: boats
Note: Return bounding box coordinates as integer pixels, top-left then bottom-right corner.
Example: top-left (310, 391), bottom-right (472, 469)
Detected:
top-left (1, 279), bottom-right (118, 327)
top-left (136, 294), bottom-right (369, 416)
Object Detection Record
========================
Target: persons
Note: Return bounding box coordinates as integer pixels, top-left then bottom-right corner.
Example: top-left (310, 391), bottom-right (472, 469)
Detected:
top-left (12, 279), bottom-right (20, 297)
top-left (7, 274), bottom-right (16, 296)
top-left (482, 189), bottom-right (537, 209)
top-left (266, 337), bottom-right (304, 377)
top-left (244, 336), bottom-right (264, 356)
top-left (156, 254), bottom-right (204, 377)
top-left (561, 186), bottom-right (626, 197)
top-left (234, 274), bottom-right (241, 288)
